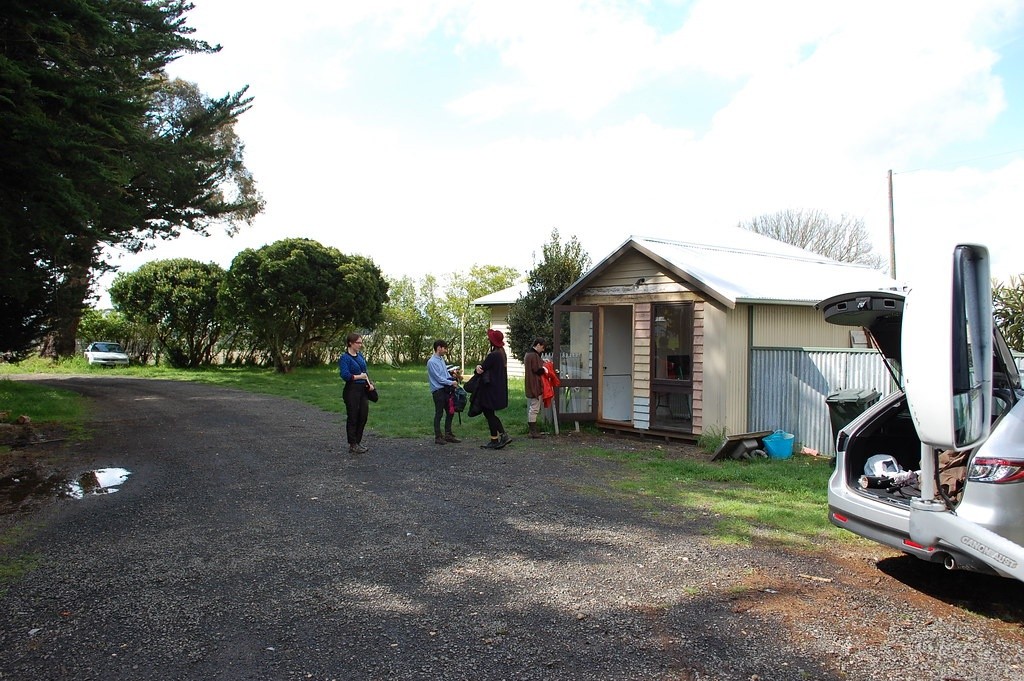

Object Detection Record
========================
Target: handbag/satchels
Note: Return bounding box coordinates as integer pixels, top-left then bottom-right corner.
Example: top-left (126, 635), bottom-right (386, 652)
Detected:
top-left (366, 386), bottom-right (378, 402)
top-left (454, 385), bottom-right (467, 412)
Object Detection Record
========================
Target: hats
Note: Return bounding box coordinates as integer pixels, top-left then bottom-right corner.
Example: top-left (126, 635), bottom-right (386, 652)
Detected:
top-left (487, 329), bottom-right (505, 347)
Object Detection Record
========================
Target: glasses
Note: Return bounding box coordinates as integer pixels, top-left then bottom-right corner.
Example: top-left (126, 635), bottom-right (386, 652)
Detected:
top-left (355, 342), bottom-right (362, 344)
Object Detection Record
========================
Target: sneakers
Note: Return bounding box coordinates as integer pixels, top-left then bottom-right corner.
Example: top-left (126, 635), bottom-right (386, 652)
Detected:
top-left (445, 432), bottom-right (461, 443)
top-left (349, 444), bottom-right (368, 454)
top-left (494, 433), bottom-right (512, 449)
top-left (435, 437), bottom-right (447, 445)
top-left (481, 438), bottom-right (499, 449)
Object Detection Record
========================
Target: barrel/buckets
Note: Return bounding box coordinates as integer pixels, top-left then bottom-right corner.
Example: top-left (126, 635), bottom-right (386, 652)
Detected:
top-left (762, 429), bottom-right (794, 461)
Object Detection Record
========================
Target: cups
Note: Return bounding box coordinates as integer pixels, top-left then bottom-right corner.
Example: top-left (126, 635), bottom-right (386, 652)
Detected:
top-left (861, 476), bottom-right (887, 490)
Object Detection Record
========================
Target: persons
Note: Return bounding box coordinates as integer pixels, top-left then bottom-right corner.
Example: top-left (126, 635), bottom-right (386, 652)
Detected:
top-left (473, 329), bottom-right (513, 449)
top-left (523, 337), bottom-right (552, 439)
top-left (427, 339), bottom-right (463, 445)
top-left (339, 334), bottom-right (375, 454)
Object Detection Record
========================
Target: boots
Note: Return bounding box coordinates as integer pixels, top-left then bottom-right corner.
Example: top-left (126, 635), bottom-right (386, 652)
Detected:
top-left (527, 421), bottom-right (544, 439)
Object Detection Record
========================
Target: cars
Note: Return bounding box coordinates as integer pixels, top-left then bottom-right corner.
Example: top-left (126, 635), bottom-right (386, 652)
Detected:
top-left (83, 342), bottom-right (131, 369)
top-left (812, 288), bottom-right (1024, 596)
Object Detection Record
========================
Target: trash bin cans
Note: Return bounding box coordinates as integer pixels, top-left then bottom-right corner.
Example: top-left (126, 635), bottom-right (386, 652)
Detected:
top-left (824, 387), bottom-right (882, 470)
